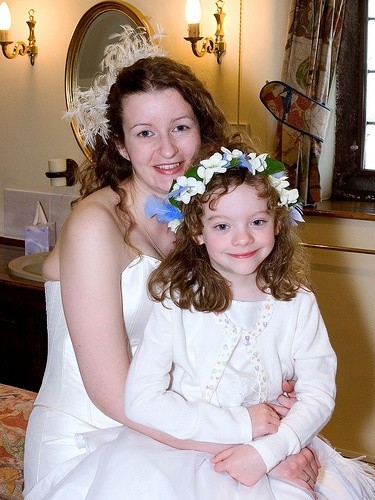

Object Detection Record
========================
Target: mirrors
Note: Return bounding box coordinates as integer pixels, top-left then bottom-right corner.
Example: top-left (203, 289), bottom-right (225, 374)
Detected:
top-left (65, 0), bottom-right (156, 169)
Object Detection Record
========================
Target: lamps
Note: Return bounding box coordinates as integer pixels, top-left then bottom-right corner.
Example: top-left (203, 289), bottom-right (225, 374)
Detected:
top-left (181, 1), bottom-right (226, 64)
top-left (0, 2), bottom-right (38, 66)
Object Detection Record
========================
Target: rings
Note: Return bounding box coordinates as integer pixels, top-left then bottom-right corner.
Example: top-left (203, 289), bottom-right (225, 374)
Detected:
top-left (305, 477), bottom-right (312, 483)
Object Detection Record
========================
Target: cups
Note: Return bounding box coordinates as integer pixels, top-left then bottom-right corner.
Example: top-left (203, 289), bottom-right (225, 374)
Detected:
top-left (48, 160), bottom-right (67, 186)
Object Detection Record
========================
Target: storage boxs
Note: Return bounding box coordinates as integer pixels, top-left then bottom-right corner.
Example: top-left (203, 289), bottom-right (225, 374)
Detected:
top-left (25, 222), bottom-right (55, 254)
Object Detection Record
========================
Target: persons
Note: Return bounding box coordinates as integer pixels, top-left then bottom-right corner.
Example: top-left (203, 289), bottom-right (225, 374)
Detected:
top-left (27, 142), bottom-right (339, 500)
top-left (22, 24), bottom-right (375, 500)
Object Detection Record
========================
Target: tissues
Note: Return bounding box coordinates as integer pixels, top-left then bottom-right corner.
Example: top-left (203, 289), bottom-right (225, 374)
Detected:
top-left (24, 201), bottom-right (55, 255)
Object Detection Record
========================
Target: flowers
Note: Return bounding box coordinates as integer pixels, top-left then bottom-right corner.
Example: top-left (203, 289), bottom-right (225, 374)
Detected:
top-left (144, 149), bottom-right (306, 236)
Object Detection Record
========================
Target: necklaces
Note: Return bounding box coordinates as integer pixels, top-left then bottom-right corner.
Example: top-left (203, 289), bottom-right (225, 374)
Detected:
top-left (129, 193), bottom-right (167, 259)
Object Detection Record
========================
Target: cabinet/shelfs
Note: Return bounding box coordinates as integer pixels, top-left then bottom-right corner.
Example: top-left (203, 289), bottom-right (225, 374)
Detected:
top-left (289, 194), bottom-right (375, 465)
